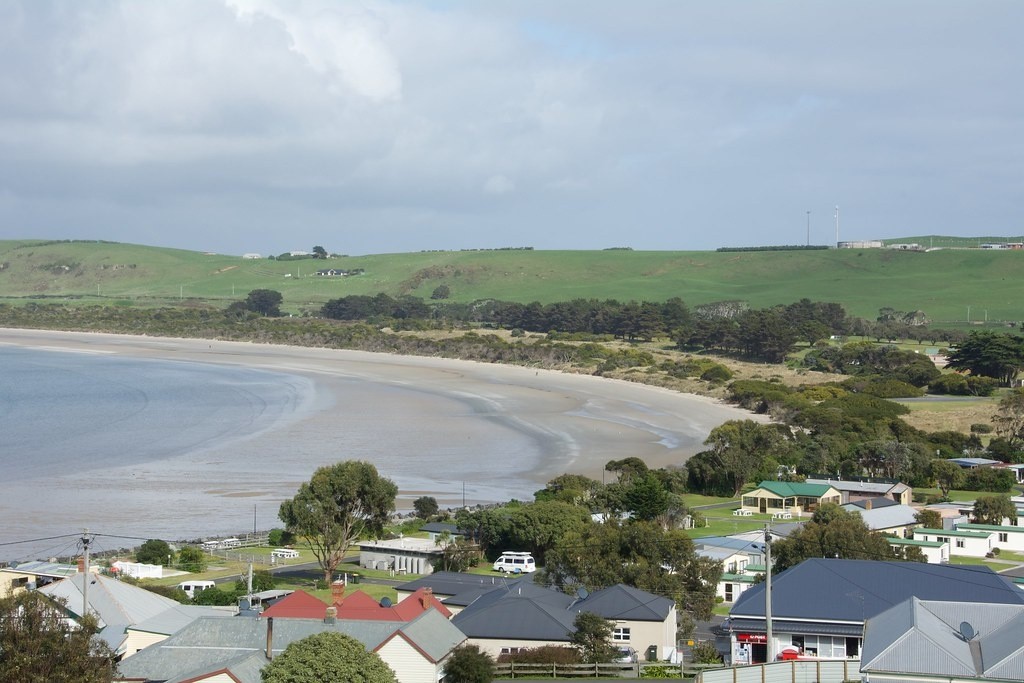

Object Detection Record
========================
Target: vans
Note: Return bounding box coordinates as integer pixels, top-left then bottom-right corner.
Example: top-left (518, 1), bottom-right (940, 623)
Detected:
top-left (493, 552), bottom-right (535, 575)
top-left (175, 581), bottom-right (215, 598)
